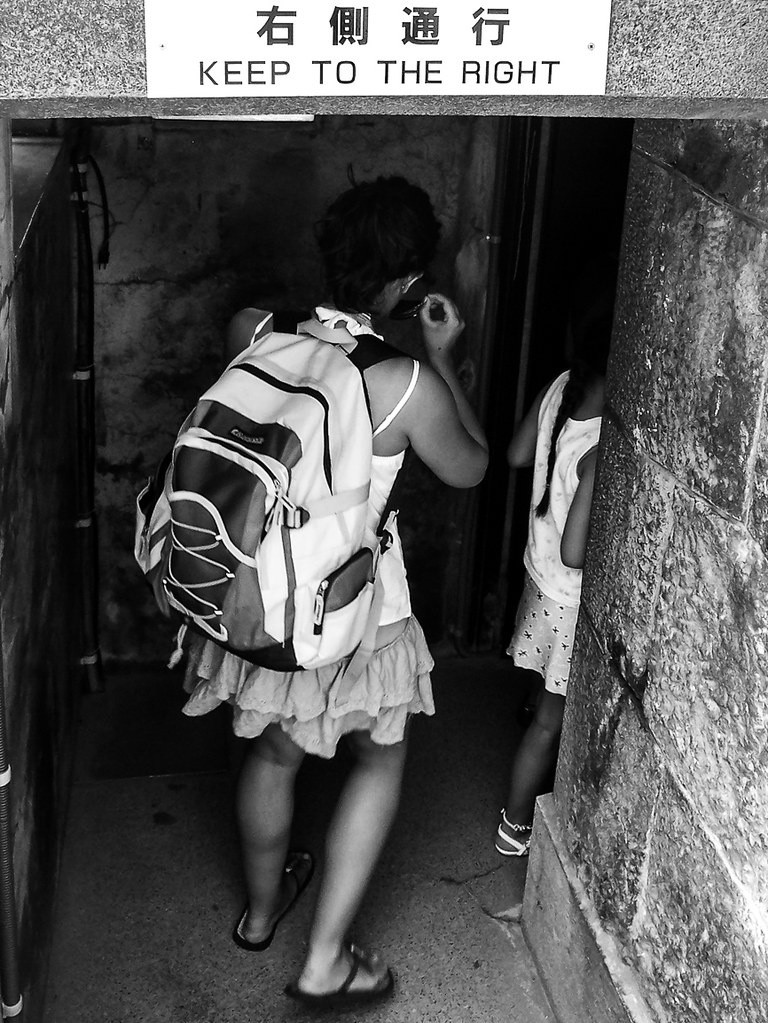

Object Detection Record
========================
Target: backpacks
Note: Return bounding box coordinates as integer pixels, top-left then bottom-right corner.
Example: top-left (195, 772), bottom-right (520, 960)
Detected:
top-left (133, 320), bottom-right (393, 705)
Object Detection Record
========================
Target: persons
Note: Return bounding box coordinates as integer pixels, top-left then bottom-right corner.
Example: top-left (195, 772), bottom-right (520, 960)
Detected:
top-left (492, 294), bottom-right (611, 858)
top-left (131, 162), bottom-right (490, 1007)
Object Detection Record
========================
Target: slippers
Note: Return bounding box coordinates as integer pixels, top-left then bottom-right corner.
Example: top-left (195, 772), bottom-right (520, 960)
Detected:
top-left (234, 850), bottom-right (316, 951)
top-left (288, 943), bottom-right (394, 1006)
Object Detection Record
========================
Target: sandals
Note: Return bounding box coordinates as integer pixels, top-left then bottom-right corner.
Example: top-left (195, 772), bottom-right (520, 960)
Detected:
top-left (494, 809), bottom-right (534, 857)
